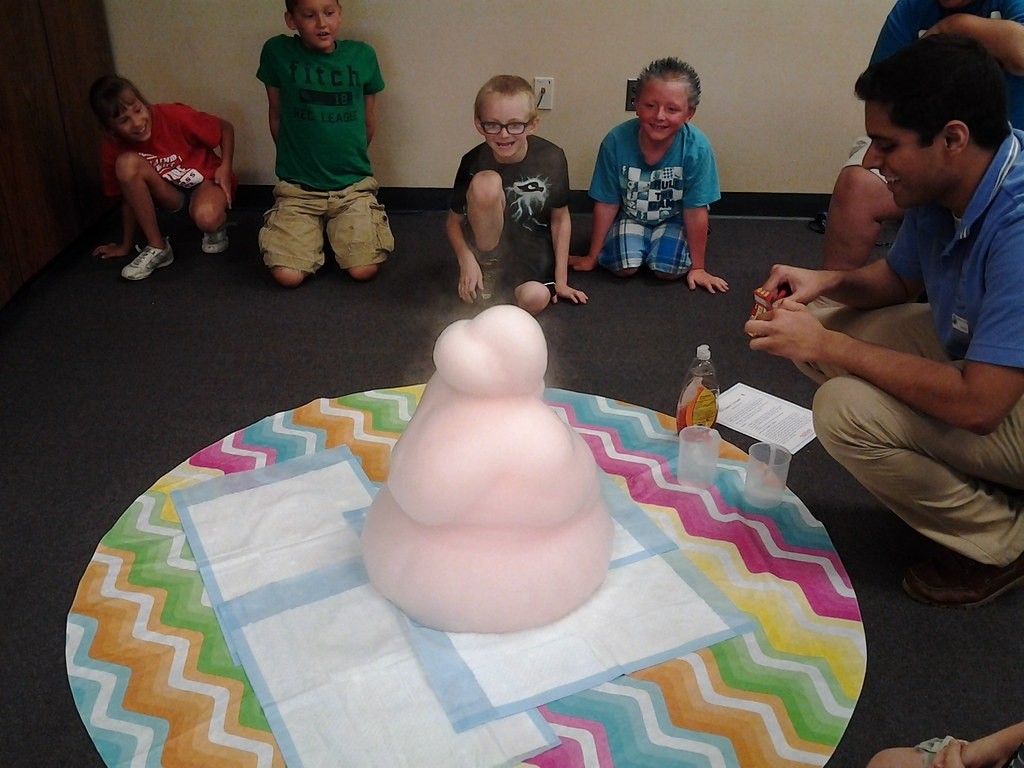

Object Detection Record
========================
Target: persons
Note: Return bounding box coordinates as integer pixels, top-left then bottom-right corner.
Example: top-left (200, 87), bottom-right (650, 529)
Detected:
top-left (89, 76), bottom-right (240, 281)
top-left (445, 73), bottom-right (591, 317)
top-left (816, 1), bottom-right (1023, 269)
top-left (255, 1), bottom-right (395, 288)
top-left (566, 56), bottom-right (730, 296)
top-left (741, 32), bottom-right (1024, 612)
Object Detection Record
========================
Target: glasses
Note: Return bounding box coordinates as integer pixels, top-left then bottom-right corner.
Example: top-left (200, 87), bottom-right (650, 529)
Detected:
top-left (477, 115), bottom-right (533, 135)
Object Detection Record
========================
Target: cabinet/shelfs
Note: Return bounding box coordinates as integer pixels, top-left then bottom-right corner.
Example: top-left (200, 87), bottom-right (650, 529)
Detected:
top-left (1, 0), bottom-right (120, 306)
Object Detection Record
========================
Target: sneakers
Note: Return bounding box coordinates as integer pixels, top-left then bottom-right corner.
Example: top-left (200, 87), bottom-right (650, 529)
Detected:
top-left (474, 260), bottom-right (499, 304)
top-left (899, 549), bottom-right (1022, 610)
top-left (201, 230), bottom-right (230, 254)
top-left (121, 237), bottom-right (175, 280)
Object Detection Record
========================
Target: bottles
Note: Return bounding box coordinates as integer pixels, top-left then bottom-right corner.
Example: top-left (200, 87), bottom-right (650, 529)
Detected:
top-left (675, 344), bottom-right (721, 443)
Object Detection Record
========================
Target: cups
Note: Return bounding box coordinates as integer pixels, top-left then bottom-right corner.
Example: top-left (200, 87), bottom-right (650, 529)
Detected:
top-left (744, 443), bottom-right (792, 510)
top-left (677, 425), bottom-right (721, 492)
